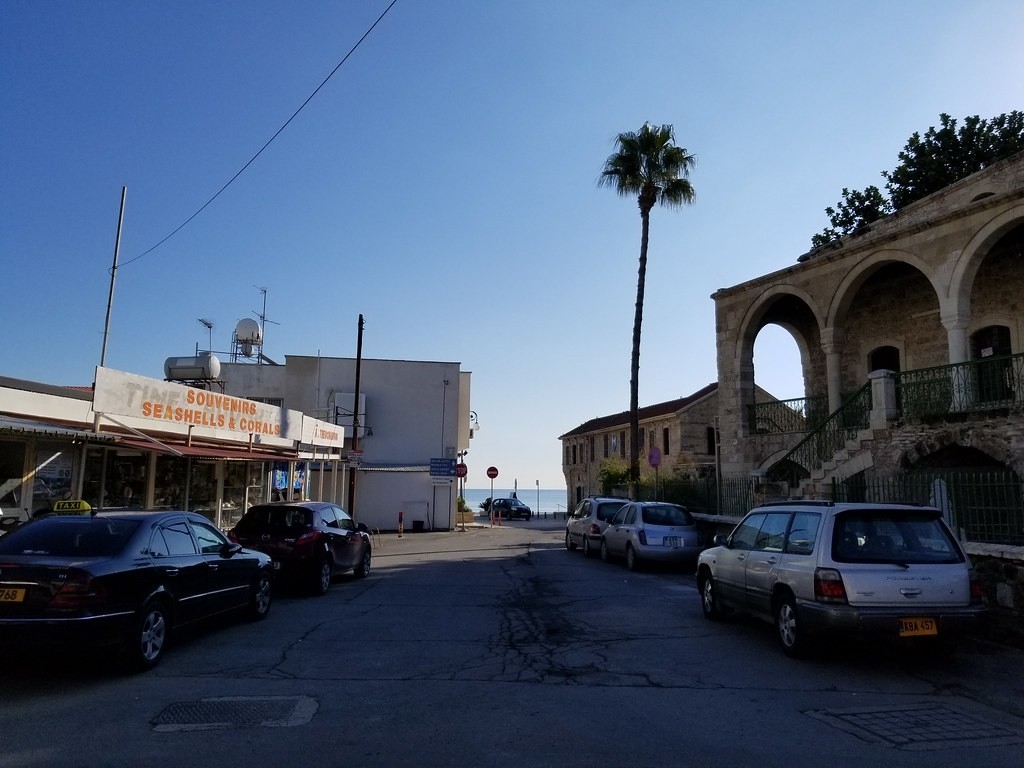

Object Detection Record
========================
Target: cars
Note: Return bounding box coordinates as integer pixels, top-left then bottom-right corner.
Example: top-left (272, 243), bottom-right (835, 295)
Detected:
top-left (564, 494), bottom-right (637, 558)
top-left (226, 499), bottom-right (373, 598)
top-left (1, 498), bottom-right (275, 672)
top-left (488, 497), bottom-right (531, 521)
top-left (600, 500), bottom-right (706, 575)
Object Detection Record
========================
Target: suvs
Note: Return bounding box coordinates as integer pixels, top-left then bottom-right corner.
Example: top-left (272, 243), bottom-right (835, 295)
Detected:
top-left (695, 498), bottom-right (989, 660)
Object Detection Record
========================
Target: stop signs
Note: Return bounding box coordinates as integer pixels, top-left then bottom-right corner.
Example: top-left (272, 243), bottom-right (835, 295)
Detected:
top-left (487, 466), bottom-right (498, 479)
top-left (456, 463), bottom-right (468, 478)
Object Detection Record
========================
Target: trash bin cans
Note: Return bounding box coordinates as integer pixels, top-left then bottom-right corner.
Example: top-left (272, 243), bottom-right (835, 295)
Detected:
top-left (413, 521), bottom-right (424, 533)
